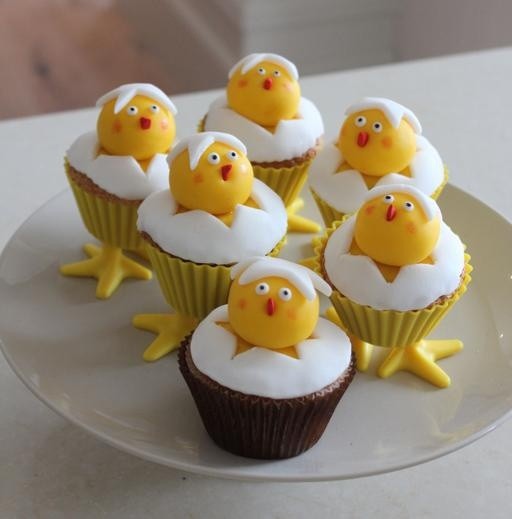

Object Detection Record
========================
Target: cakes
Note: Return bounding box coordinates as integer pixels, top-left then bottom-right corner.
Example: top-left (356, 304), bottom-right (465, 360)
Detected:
top-left (61, 83), bottom-right (172, 300)
top-left (178, 256), bottom-right (356, 459)
top-left (308, 97), bottom-right (448, 229)
top-left (197, 52), bottom-right (325, 242)
top-left (129, 130), bottom-right (287, 362)
top-left (314, 182), bottom-right (474, 387)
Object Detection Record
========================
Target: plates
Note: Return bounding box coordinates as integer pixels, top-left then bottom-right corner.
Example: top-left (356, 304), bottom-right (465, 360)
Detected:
top-left (0, 129), bottom-right (512, 482)
